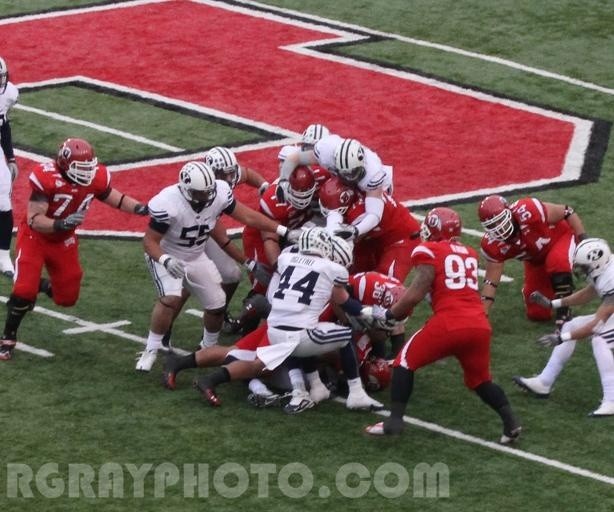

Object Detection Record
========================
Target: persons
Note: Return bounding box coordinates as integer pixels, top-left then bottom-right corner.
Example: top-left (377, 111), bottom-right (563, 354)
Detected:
top-left (0, 58), bottom-right (614, 445)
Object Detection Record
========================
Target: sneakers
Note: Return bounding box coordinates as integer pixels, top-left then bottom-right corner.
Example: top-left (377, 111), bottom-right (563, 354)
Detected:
top-left (7, 158), bottom-right (18, 182)
top-left (365, 419), bottom-right (404, 435)
top-left (0, 249), bottom-right (14, 277)
top-left (587, 400), bottom-right (614, 418)
top-left (0, 336), bottom-right (16, 360)
top-left (512, 376), bottom-right (551, 399)
top-left (136, 311), bottom-right (384, 415)
top-left (500, 427), bottom-right (521, 443)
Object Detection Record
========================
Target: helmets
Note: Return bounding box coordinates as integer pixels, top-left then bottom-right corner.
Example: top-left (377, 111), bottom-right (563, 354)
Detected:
top-left (56, 138), bottom-right (99, 187)
top-left (572, 238), bottom-right (611, 285)
top-left (420, 207), bottom-right (461, 242)
top-left (179, 146), bottom-right (238, 208)
top-left (377, 286), bottom-right (413, 330)
top-left (479, 195), bottom-right (514, 242)
top-left (365, 358), bottom-right (392, 392)
top-left (0, 57), bottom-right (8, 95)
top-left (278, 124), bottom-right (366, 269)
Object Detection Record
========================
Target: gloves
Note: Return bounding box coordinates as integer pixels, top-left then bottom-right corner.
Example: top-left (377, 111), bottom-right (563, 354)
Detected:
top-left (159, 254), bottom-right (186, 279)
top-left (536, 334), bottom-right (562, 348)
top-left (54, 214), bottom-right (85, 232)
top-left (135, 204), bottom-right (149, 216)
top-left (357, 304), bottom-right (388, 321)
top-left (241, 257), bottom-right (272, 285)
top-left (530, 291), bottom-right (552, 307)
